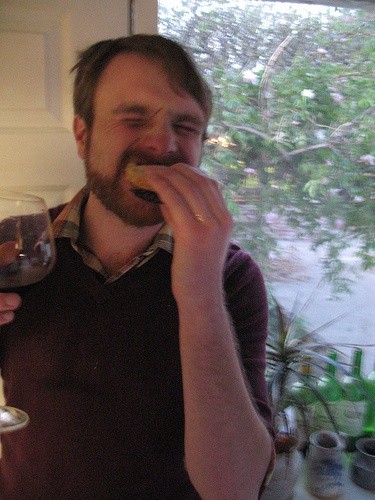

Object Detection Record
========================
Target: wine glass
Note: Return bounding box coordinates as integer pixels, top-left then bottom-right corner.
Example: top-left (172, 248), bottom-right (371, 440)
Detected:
top-left (0, 191), bottom-right (55, 432)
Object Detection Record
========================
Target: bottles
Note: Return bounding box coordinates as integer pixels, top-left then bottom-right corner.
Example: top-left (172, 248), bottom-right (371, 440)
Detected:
top-left (342, 347), bottom-right (367, 453)
top-left (315, 350), bottom-right (342, 432)
top-left (367, 357), bottom-right (375, 441)
top-left (307, 431), bottom-right (346, 500)
top-left (291, 358), bottom-right (317, 453)
top-left (350, 437), bottom-right (375, 498)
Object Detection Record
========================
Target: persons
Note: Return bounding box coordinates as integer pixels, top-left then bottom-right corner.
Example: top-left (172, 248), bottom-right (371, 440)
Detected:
top-left (1, 35), bottom-right (274, 496)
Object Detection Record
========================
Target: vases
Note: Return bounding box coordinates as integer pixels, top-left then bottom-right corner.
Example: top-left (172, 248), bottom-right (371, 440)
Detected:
top-left (258, 433), bottom-right (302, 500)
top-left (350, 438), bottom-right (375, 489)
top-left (305, 430), bottom-right (347, 498)
